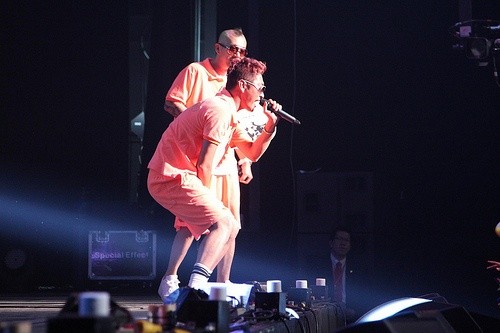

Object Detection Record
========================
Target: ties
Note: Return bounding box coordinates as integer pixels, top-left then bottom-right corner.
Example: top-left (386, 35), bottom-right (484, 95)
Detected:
top-left (336, 262), bottom-right (343, 302)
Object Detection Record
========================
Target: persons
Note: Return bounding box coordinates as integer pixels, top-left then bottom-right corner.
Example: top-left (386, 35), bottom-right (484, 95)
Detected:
top-left (157, 28), bottom-right (253, 300)
top-left (146, 57), bottom-right (278, 290)
top-left (310, 227), bottom-right (375, 325)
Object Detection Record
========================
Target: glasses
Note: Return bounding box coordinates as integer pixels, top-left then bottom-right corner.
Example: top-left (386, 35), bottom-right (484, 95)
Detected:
top-left (237, 79), bottom-right (266, 92)
top-left (216, 42), bottom-right (248, 57)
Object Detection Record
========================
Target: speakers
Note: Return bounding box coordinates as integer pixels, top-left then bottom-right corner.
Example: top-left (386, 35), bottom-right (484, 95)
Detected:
top-left (46, 310), bottom-right (122, 333)
top-left (310, 285), bottom-right (330, 300)
top-left (253, 292), bottom-right (286, 317)
top-left (175, 299), bottom-right (230, 333)
top-left (288, 288), bottom-right (311, 309)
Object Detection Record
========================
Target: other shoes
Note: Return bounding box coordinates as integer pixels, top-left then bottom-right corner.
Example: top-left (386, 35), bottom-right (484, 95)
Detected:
top-left (158, 276), bottom-right (181, 298)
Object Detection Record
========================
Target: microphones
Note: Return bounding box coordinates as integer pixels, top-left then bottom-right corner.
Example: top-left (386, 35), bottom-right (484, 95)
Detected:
top-left (259, 98), bottom-right (301, 124)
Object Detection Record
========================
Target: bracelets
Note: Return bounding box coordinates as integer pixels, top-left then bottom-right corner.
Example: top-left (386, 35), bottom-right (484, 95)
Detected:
top-left (238, 157), bottom-right (253, 166)
top-left (263, 126), bottom-right (277, 134)
